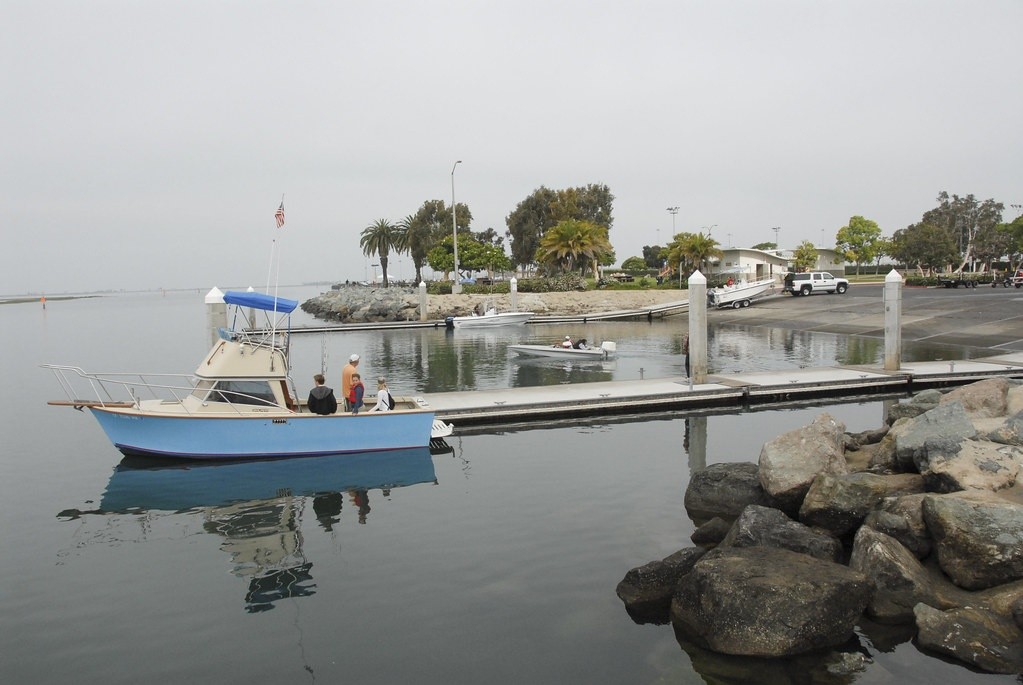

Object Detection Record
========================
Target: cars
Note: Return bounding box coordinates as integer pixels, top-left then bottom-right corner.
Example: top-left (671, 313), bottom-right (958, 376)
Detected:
top-left (991, 269), bottom-right (1023, 288)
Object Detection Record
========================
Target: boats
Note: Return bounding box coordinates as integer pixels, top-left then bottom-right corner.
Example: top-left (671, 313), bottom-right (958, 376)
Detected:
top-left (38, 285), bottom-right (455, 462)
top-left (444, 301), bottom-right (534, 330)
top-left (505, 340), bottom-right (616, 361)
top-left (95, 447), bottom-right (440, 613)
top-left (705, 274), bottom-right (776, 308)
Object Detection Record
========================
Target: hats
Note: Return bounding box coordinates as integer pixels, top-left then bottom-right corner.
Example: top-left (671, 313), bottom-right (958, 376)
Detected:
top-left (565, 336), bottom-right (570, 339)
top-left (349, 354), bottom-right (359, 362)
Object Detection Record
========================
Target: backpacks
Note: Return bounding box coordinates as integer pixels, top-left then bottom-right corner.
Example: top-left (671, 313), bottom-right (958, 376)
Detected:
top-left (382, 393), bottom-right (395, 410)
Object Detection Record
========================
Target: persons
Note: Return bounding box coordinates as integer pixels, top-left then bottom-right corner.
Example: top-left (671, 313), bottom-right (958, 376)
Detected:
top-left (785, 273), bottom-right (790, 295)
top-left (349, 374), bottom-right (366, 414)
top-left (727, 276), bottom-right (734, 286)
top-left (369, 377), bottom-right (391, 413)
top-left (342, 354), bottom-right (359, 412)
top-left (307, 375), bottom-right (337, 415)
top-left (555, 336), bottom-right (590, 351)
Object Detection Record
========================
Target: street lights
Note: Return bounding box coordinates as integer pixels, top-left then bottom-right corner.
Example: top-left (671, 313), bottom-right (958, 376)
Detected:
top-left (666, 206), bottom-right (680, 242)
top-left (450, 160), bottom-right (463, 292)
top-left (772, 226), bottom-right (780, 249)
top-left (701, 224), bottom-right (718, 240)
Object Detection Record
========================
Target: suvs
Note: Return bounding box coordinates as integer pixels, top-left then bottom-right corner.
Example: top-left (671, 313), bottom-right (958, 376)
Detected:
top-left (780, 271), bottom-right (851, 297)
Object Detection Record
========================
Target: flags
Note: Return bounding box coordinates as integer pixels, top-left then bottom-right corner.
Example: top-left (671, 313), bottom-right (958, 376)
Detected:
top-left (276, 198), bottom-right (285, 228)
